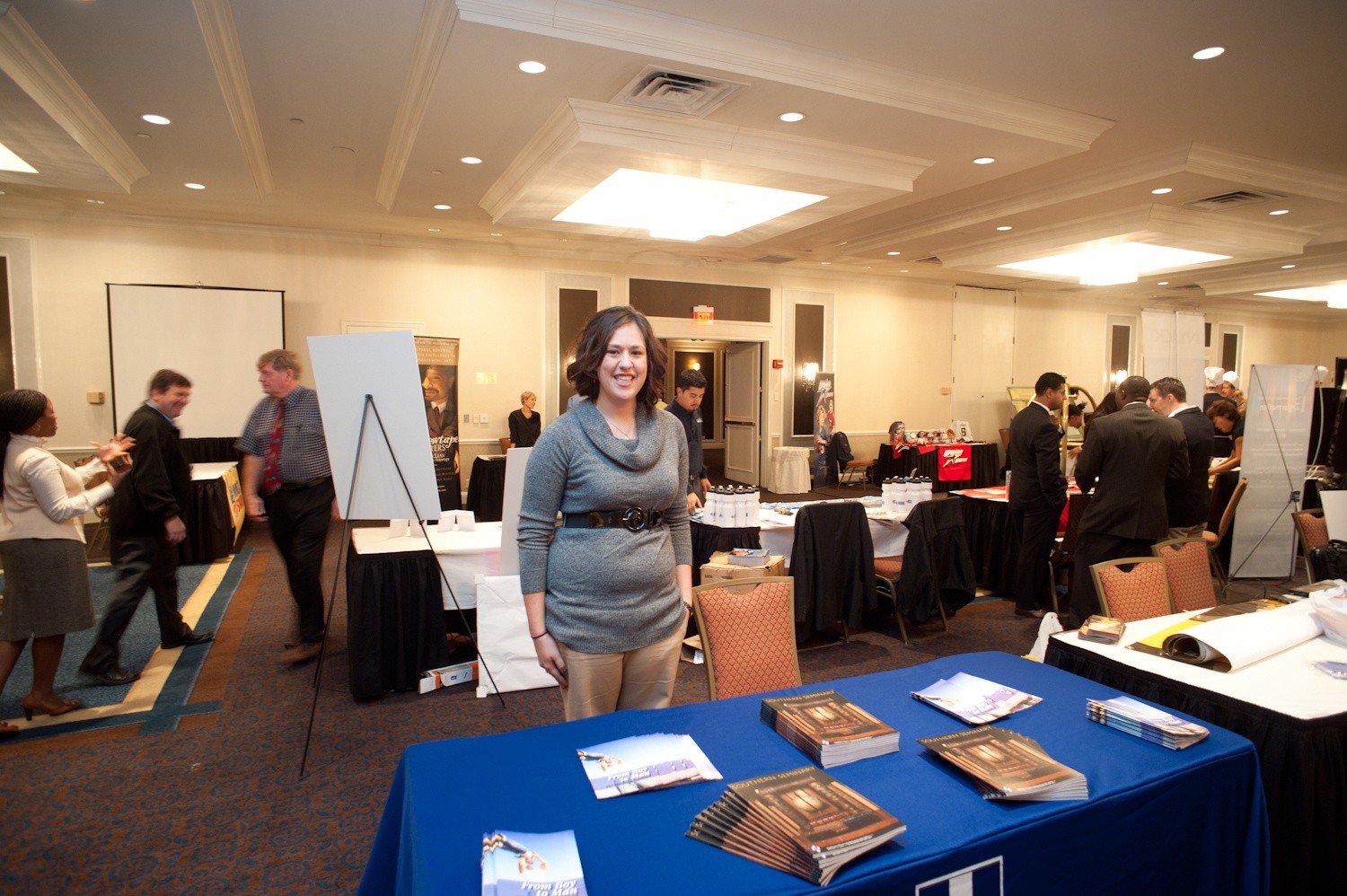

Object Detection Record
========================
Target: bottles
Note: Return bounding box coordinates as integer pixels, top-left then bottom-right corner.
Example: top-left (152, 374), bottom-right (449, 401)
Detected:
top-left (882, 475), bottom-right (933, 516)
top-left (702, 483), bottom-right (761, 529)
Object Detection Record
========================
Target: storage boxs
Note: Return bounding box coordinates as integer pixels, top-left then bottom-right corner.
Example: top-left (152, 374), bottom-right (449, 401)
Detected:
top-left (418, 660), bottom-right (478, 695)
top-left (699, 555), bottom-right (785, 586)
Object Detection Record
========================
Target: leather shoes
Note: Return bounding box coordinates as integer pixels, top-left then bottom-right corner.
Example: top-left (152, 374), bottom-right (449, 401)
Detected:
top-left (164, 630), bottom-right (214, 649)
top-left (87, 666), bottom-right (140, 685)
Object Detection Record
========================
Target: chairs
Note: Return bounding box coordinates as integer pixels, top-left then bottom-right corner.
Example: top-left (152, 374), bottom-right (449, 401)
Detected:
top-left (874, 497), bottom-right (977, 646)
top-left (692, 575), bottom-right (802, 704)
top-left (74, 457), bottom-right (108, 558)
top-left (1202, 477), bottom-right (1247, 598)
top-left (1290, 509), bottom-right (1329, 585)
top-left (1048, 494), bottom-right (1089, 614)
top-left (1089, 557), bottom-right (1173, 622)
top-left (832, 431), bottom-right (874, 492)
top-left (789, 501), bottom-right (879, 652)
top-left (1151, 537), bottom-right (1217, 613)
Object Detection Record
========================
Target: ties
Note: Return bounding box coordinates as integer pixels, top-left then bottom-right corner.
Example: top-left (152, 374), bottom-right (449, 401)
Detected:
top-left (264, 399), bottom-right (287, 495)
top-left (434, 407), bottom-right (439, 420)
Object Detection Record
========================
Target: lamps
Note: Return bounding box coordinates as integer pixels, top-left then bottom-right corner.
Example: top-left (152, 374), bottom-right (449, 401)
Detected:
top-left (476, 96), bottom-right (936, 250)
top-left (935, 202), bottom-right (1347, 309)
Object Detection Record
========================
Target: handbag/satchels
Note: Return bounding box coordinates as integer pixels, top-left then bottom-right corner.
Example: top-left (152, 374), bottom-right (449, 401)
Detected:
top-left (1310, 539), bottom-right (1347, 581)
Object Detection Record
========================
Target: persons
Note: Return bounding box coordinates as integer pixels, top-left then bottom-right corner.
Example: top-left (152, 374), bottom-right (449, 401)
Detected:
top-left (0, 389), bottom-right (136, 742)
top-left (816, 399), bottom-right (835, 471)
top-left (1066, 376), bottom-right (1191, 628)
top-left (515, 305), bottom-right (694, 723)
top-left (653, 388), bottom-right (669, 410)
top-left (78, 369), bottom-right (215, 681)
top-left (662, 369), bottom-right (711, 516)
top-left (566, 392), bottom-right (589, 410)
top-left (233, 349), bottom-right (336, 664)
top-left (508, 391), bottom-right (541, 448)
top-left (421, 365), bottom-right (460, 474)
top-left (1065, 365), bottom-right (1329, 551)
top-left (1004, 372), bottom-right (1068, 618)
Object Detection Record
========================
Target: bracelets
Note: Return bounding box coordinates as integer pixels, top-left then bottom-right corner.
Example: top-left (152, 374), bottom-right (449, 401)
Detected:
top-left (531, 631), bottom-right (549, 639)
top-left (683, 601), bottom-right (694, 617)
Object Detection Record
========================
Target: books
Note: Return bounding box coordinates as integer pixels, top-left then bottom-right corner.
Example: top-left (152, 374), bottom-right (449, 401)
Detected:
top-left (684, 765), bottom-right (907, 886)
top-left (576, 732), bottom-right (724, 801)
top-left (759, 689), bottom-right (901, 769)
top-left (909, 671), bottom-right (1211, 801)
top-left (480, 829), bottom-right (588, 896)
top-left (1077, 614), bottom-right (1126, 644)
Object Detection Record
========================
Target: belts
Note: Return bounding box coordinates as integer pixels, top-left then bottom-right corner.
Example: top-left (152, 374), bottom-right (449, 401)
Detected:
top-left (689, 473), bottom-right (701, 479)
top-left (563, 506), bottom-right (664, 531)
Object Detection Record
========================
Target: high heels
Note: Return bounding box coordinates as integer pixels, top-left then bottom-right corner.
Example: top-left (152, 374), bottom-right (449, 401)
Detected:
top-left (22, 691), bottom-right (82, 721)
top-left (0, 720), bottom-right (18, 738)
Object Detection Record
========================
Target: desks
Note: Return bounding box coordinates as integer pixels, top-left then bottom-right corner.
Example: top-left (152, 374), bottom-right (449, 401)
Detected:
top-left (688, 498), bottom-right (910, 589)
top-left (767, 446), bottom-right (812, 494)
top-left (353, 651), bottom-right (1269, 896)
top-left (346, 522), bottom-right (502, 704)
top-left (875, 443), bottom-right (1001, 492)
top-left (193, 462), bottom-right (246, 560)
top-left (948, 481), bottom-right (1081, 590)
top-left (466, 455), bottom-right (506, 523)
top-left (1043, 592), bottom-right (1347, 896)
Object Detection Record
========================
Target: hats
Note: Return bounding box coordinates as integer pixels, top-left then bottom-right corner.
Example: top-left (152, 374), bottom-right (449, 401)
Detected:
top-left (1315, 365), bottom-right (1329, 383)
top-left (1222, 371), bottom-right (1241, 390)
top-left (1205, 367), bottom-right (1224, 388)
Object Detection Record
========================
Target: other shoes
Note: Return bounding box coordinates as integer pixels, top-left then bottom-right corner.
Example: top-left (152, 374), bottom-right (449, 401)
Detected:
top-left (1014, 605), bottom-right (1046, 618)
top-left (279, 643), bottom-right (320, 662)
top-left (282, 631), bottom-right (304, 649)
